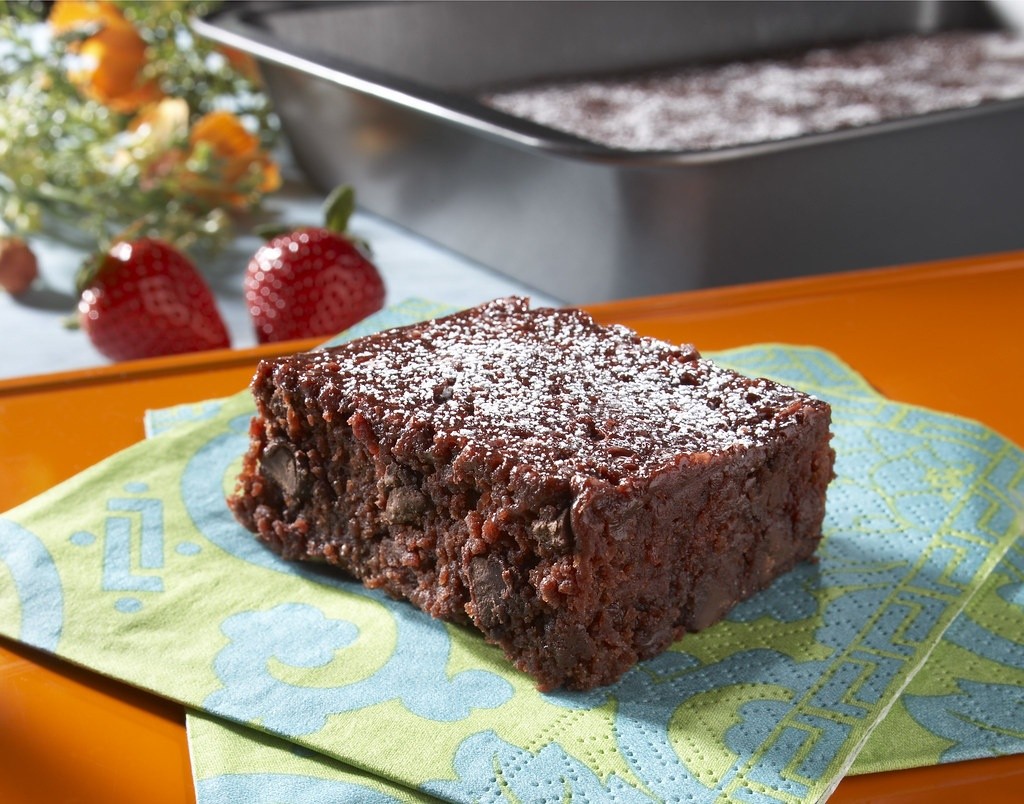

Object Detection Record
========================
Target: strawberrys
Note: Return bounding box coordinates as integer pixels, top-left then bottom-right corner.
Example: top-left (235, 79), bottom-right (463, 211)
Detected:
top-left (75, 236), bottom-right (228, 360)
top-left (241, 185), bottom-right (385, 343)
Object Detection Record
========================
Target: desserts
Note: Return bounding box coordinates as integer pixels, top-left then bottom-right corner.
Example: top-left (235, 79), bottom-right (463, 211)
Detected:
top-left (229, 297), bottom-right (833, 689)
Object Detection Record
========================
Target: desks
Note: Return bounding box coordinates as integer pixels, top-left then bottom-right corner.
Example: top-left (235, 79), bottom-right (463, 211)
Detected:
top-left (0, 250), bottom-right (1024, 804)
